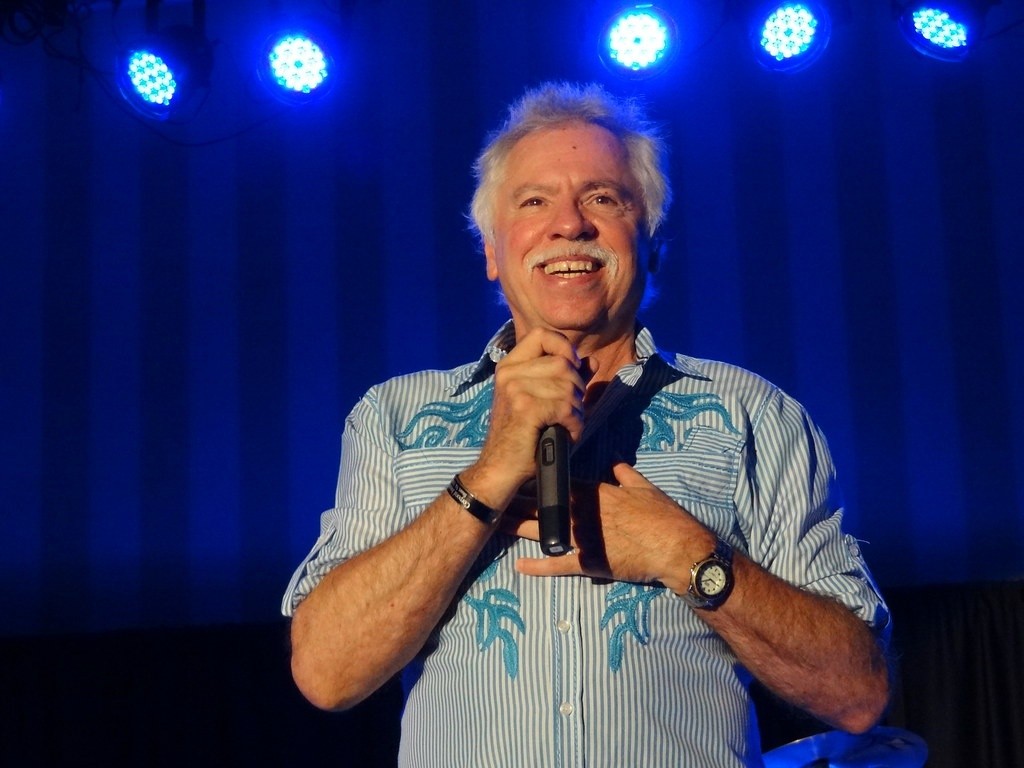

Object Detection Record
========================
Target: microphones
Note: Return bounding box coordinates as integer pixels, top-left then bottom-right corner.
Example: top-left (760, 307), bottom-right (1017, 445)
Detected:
top-left (536, 423), bottom-right (573, 558)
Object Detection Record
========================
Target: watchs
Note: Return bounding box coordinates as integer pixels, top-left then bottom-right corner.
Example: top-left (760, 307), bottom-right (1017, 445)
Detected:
top-left (679, 535), bottom-right (735, 609)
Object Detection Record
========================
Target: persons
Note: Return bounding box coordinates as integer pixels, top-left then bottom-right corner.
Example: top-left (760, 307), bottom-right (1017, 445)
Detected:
top-left (279, 82), bottom-right (894, 768)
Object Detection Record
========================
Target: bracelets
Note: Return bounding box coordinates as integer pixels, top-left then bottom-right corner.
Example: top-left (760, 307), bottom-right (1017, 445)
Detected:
top-left (446, 473), bottom-right (501, 527)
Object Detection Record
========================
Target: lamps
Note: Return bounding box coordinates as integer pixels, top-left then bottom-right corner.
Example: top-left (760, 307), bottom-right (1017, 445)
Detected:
top-left (251, 0), bottom-right (340, 106)
top-left (117, 0), bottom-right (215, 116)
top-left (899, 0), bottom-right (995, 63)
top-left (596, 0), bottom-right (682, 86)
top-left (724, 0), bottom-right (835, 77)
